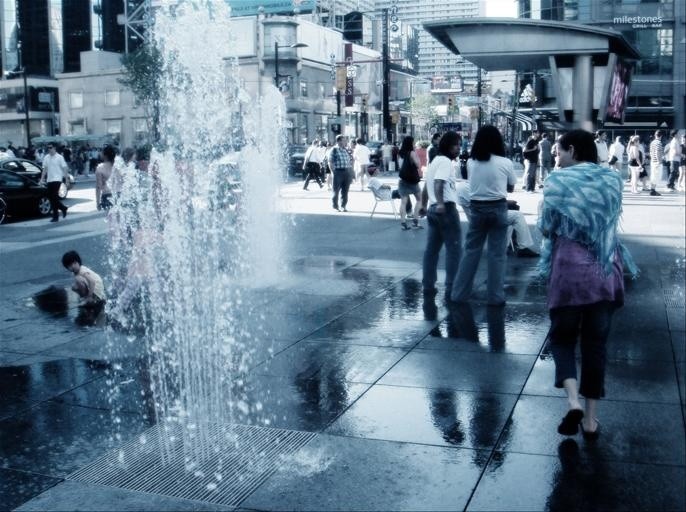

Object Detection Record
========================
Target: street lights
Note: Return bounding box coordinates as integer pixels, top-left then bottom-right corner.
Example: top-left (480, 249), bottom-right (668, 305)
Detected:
top-left (270, 39), bottom-right (308, 88)
top-left (407, 79), bottom-right (427, 135)
top-left (16, 40), bottom-right (22, 72)
top-left (2, 66), bottom-right (33, 160)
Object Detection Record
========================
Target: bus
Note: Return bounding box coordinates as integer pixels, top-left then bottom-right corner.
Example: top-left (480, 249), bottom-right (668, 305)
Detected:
top-left (435, 122), bottom-right (472, 139)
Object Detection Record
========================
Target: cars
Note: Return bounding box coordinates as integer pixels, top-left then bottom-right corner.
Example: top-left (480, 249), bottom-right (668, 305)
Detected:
top-left (288, 145), bottom-right (326, 178)
top-left (0, 158), bottom-right (75, 200)
top-left (208, 152), bottom-right (240, 182)
top-left (363, 141), bottom-right (382, 166)
top-left (0, 168), bottom-right (50, 222)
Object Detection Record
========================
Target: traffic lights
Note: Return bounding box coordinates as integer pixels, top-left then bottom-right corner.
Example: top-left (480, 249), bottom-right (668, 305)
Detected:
top-left (447, 97), bottom-right (454, 111)
top-left (360, 95), bottom-right (368, 111)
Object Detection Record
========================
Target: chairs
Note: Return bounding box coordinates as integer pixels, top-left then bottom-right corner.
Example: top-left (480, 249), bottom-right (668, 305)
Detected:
top-left (368, 181), bottom-right (398, 221)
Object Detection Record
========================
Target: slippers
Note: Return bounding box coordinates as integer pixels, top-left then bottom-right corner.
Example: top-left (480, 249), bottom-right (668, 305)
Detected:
top-left (557, 407), bottom-right (602, 439)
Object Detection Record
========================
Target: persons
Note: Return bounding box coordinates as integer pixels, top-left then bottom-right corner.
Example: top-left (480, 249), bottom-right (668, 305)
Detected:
top-left (595, 130), bottom-right (625, 170)
top-left (61, 251), bottom-right (104, 306)
top-left (664, 130), bottom-right (686, 191)
top-left (650, 130), bottom-right (663, 196)
top-left (398, 136), bottom-right (424, 230)
top-left (419, 132), bottom-right (463, 317)
top-left (304, 141), bottom-right (331, 190)
top-left (451, 124), bottom-right (517, 308)
top-left (459, 165), bottom-right (538, 257)
top-left (380, 140), bottom-right (400, 174)
top-left (505, 130), bottom-right (563, 193)
top-left (420, 133), bottom-right (441, 216)
top-left (350, 137), bottom-right (371, 190)
top-left (627, 135), bottom-right (650, 194)
top-left (328, 135), bottom-right (354, 212)
top-left (110, 143), bottom-right (165, 329)
top-left (0, 143), bottom-right (151, 216)
top-left (458, 145), bottom-right (469, 179)
top-left (538, 130), bottom-right (624, 441)
